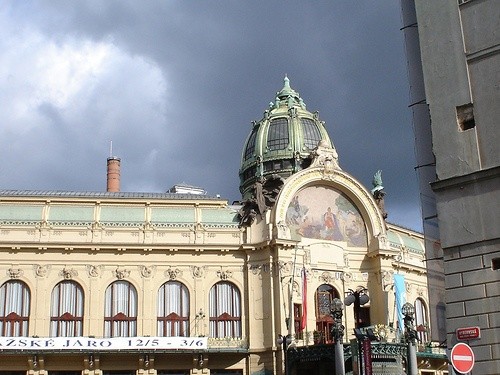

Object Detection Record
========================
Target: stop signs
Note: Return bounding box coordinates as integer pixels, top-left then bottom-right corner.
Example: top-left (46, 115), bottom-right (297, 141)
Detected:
top-left (450, 342), bottom-right (475, 374)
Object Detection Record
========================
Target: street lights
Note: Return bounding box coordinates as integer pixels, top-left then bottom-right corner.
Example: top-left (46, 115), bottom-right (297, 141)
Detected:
top-left (277, 333), bottom-right (291, 375)
top-left (344, 287), bottom-right (370, 375)
